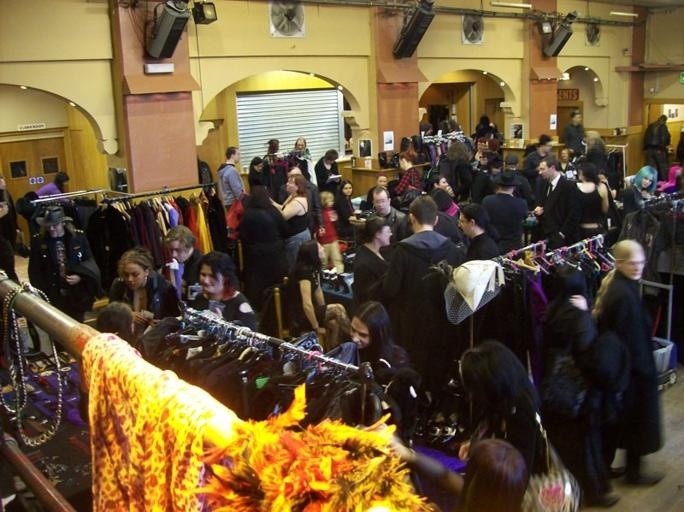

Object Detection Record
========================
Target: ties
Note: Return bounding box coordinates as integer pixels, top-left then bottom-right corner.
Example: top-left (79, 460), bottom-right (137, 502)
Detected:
top-left (547, 182), bottom-right (553, 201)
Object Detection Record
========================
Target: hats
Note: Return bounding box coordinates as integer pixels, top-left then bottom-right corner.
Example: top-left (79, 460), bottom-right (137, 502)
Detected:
top-left (35, 206), bottom-right (74, 226)
top-left (493, 171), bottom-right (523, 186)
top-left (15, 190), bottom-right (39, 222)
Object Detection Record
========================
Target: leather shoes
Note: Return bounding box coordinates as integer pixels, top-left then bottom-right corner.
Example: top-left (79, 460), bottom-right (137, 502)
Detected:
top-left (620, 465), bottom-right (667, 487)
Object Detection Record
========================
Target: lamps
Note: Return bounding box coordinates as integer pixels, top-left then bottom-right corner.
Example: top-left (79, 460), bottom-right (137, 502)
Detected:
top-left (534, 16), bottom-right (553, 35)
top-left (191, 0), bottom-right (217, 25)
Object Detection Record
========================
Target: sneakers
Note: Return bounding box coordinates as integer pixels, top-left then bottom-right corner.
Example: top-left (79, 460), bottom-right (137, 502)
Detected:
top-left (581, 468), bottom-right (623, 508)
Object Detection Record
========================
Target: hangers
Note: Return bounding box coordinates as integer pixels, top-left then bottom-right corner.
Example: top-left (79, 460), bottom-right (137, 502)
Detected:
top-left (639, 188), bottom-right (684, 218)
top-left (480, 230), bottom-right (621, 282)
top-left (420, 128), bottom-right (466, 149)
top-left (29, 190), bottom-right (94, 209)
top-left (94, 180), bottom-right (187, 215)
top-left (141, 299), bottom-right (393, 419)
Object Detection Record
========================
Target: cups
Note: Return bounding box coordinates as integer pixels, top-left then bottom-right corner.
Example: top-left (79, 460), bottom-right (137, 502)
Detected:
top-left (208, 300), bottom-right (226, 314)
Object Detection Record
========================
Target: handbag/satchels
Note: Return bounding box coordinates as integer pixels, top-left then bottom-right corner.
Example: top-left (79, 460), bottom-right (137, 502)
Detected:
top-left (600, 180), bottom-right (622, 229)
top-left (14, 227), bottom-right (30, 258)
top-left (520, 411), bottom-right (582, 512)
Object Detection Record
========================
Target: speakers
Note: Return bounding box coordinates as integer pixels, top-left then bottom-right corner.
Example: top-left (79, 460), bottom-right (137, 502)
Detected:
top-left (544, 26), bottom-right (574, 59)
top-left (145, 6), bottom-right (191, 60)
top-left (393, 9), bottom-right (436, 57)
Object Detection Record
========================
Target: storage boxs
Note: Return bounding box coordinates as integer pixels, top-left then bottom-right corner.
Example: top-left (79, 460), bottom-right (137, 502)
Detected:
top-left (651, 336), bottom-right (674, 374)
